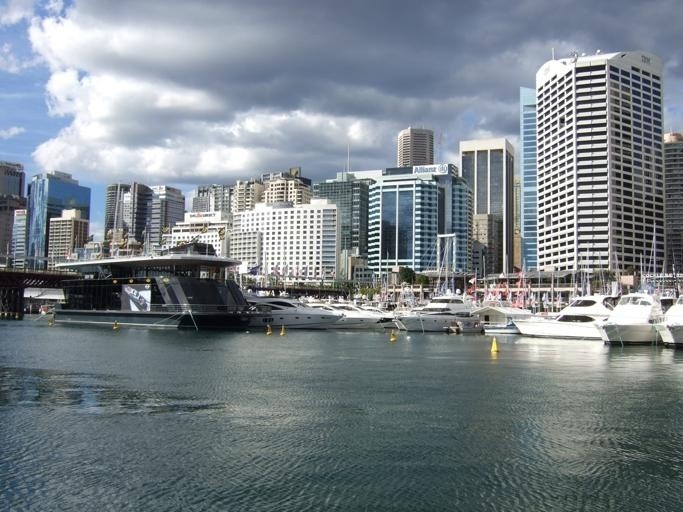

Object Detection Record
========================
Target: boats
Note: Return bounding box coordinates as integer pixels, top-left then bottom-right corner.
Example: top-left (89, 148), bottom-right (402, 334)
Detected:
top-left (50, 241), bottom-right (254, 336)
top-left (243, 242), bottom-right (540, 335)
top-left (651, 291), bottom-right (683, 348)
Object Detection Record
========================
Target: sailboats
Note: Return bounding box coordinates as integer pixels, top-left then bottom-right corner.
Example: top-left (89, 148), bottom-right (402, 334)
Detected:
top-left (592, 221), bottom-right (666, 346)
top-left (511, 245), bottom-right (618, 340)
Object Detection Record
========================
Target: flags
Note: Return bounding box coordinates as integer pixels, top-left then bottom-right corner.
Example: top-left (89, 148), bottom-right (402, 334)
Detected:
top-left (248, 263), bottom-right (344, 280)
top-left (121, 225), bottom-right (225, 247)
